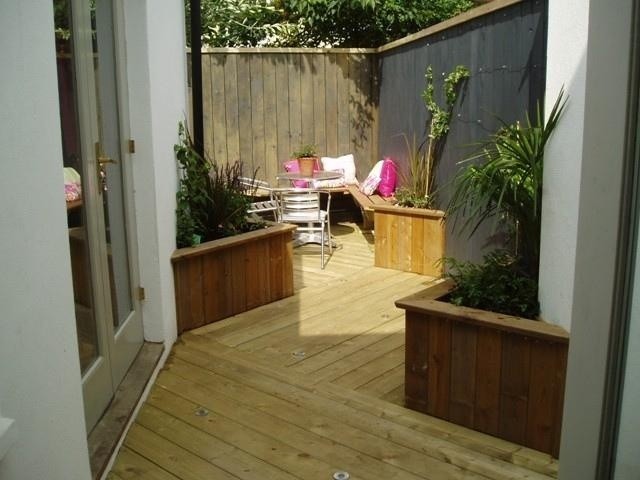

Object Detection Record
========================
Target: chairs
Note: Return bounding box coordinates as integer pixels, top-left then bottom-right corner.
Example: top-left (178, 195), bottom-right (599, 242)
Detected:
top-left (236, 177), bottom-right (333, 270)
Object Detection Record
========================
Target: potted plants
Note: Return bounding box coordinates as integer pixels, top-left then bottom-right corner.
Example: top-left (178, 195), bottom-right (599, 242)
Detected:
top-left (290, 140), bottom-right (320, 176)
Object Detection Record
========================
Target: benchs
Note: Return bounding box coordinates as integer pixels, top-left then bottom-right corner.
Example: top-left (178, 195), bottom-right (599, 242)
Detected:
top-left (279, 183), bottom-right (397, 230)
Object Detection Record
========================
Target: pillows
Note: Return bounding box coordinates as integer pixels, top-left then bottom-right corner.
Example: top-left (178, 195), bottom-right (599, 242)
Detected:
top-left (282, 153), bottom-right (398, 199)
top-left (64, 166), bottom-right (82, 202)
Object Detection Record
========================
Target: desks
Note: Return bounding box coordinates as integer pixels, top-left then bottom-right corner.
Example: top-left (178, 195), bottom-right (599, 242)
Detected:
top-left (276, 171), bottom-right (343, 251)
